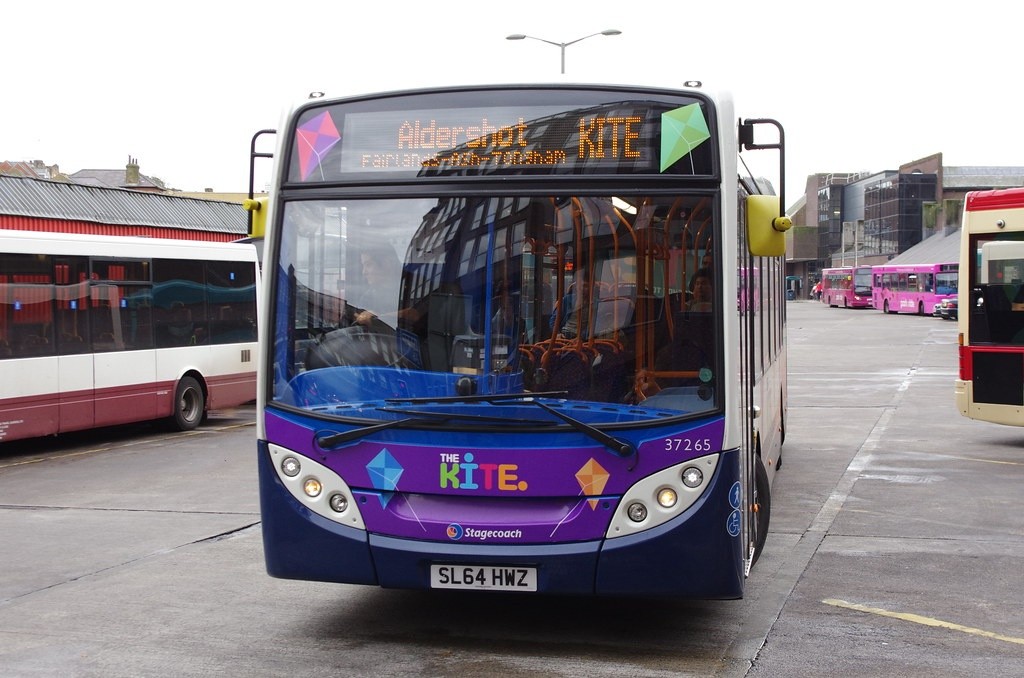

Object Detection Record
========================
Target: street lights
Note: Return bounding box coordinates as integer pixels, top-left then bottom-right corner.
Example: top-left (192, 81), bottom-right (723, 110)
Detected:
top-left (509, 30), bottom-right (624, 77)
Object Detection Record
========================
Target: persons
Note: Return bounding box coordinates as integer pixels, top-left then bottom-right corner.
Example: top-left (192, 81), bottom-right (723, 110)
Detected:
top-left (701, 252), bottom-right (711, 267)
top-left (948, 281), bottom-right (955, 288)
top-left (595, 313), bottom-right (628, 348)
top-left (340, 246), bottom-right (447, 372)
top-left (811, 282), bottom-right (816, 299)
top-left (192, 323), bottom-right (211, 345)
top-left (815, 281), bottom-right (822, 301)
top-left (547, 271), bottom-right (598, 341)
top-left (683, 268), bottom-right (712, 312)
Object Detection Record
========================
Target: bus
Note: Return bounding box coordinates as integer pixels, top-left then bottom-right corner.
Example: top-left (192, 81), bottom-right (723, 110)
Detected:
top-left (5, 229), bottom-right (258, 460)
top-left (957, 189), bottom-right (1023, 427)
top-left (822, 265), bottom-right (872, 308)
top-left (249, 79), bottom-right (793, 613)
top-left (871, 263), bottom-right (959, 319)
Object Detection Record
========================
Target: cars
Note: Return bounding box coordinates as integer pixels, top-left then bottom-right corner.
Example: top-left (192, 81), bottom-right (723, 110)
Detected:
top-left (940, 297), bottom-right (958, 319)
